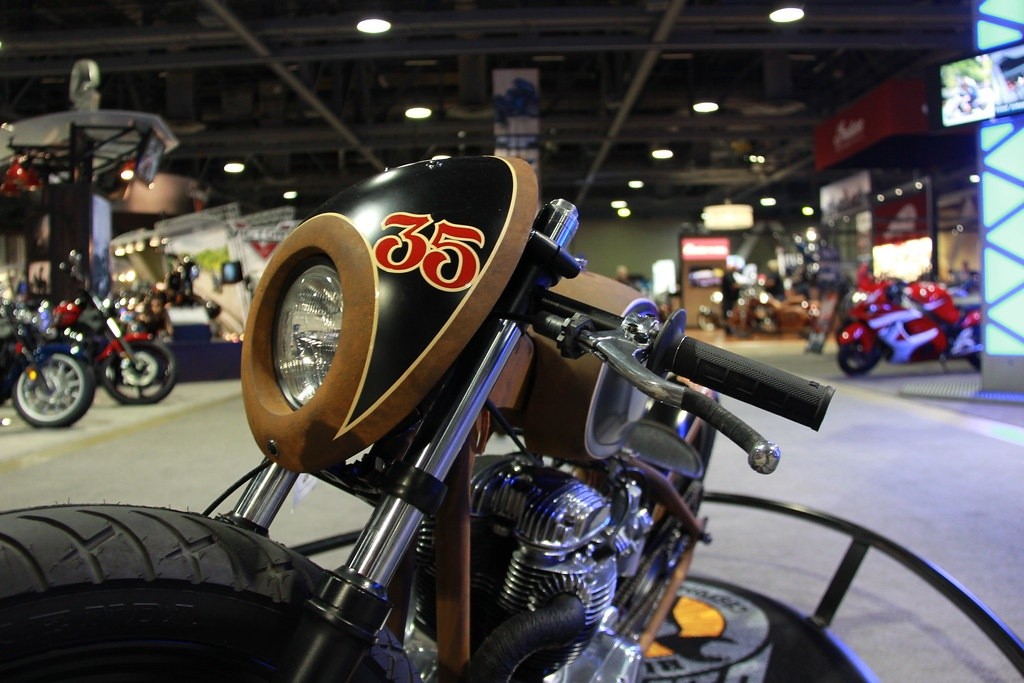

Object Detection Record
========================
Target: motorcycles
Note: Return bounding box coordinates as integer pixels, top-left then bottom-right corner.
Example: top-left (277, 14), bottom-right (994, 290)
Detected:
top-left (943, 93), bottom-right (988, 122)
top-left (0, 243), bottom-right (222, 428)
top-left (836, 259), bottom-right (985, 377)
top-left (1, 155), bottom-right (1024, 683)
top-left (695, 230), bottom-right (857, 356)
